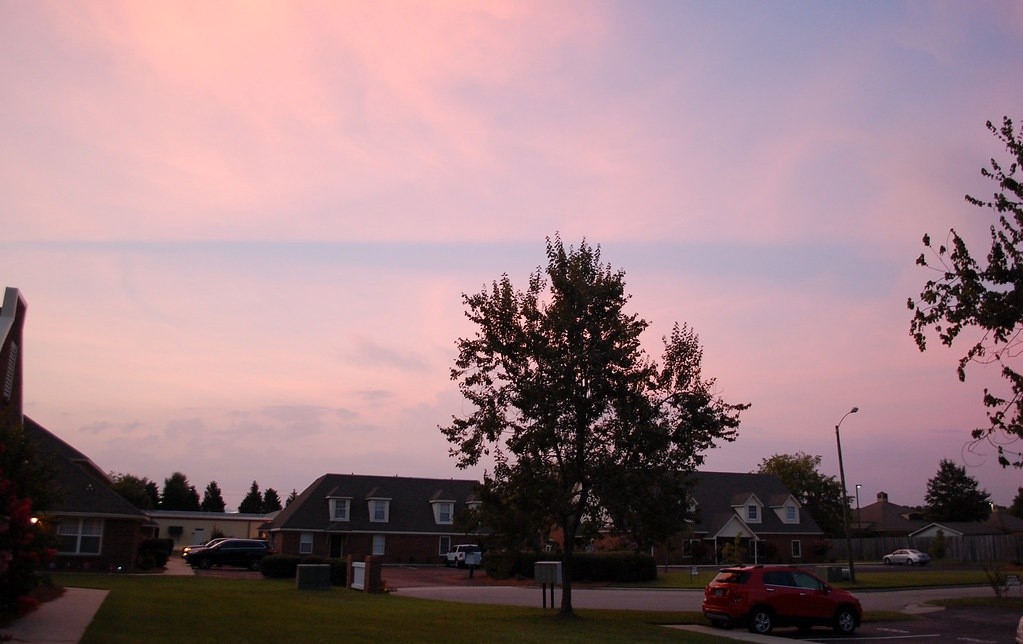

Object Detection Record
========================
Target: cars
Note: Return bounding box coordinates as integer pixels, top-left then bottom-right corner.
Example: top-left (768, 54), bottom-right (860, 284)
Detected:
top-left (181, 538), bottom-right (273, 570)
top-left (702, 564), bottom-right (864, 635)
top-left (882, 549), bottom-right (931, 566)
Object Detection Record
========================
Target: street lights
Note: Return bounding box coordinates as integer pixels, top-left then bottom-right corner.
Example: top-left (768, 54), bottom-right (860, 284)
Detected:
top-left (835, 406), bottom-right (860, 585)
top-left (854, 482), bottom-right (865, 566)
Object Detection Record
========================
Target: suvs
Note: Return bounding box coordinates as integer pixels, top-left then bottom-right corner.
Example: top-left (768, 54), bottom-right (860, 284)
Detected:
top-left (442, 543), bottom-right (481, 568)
